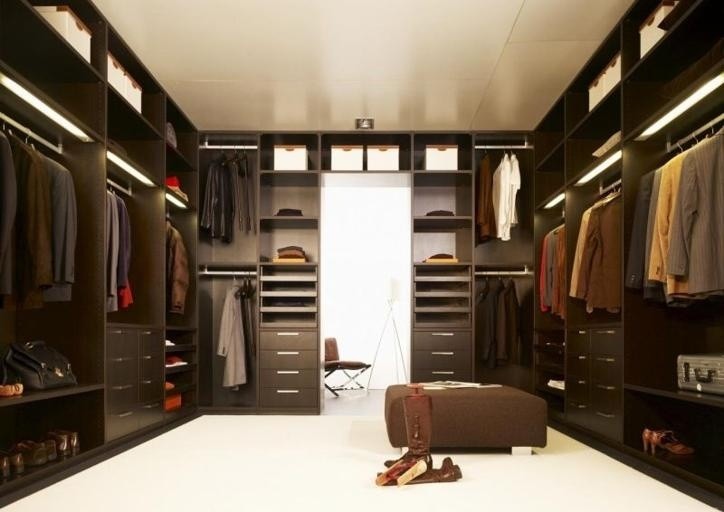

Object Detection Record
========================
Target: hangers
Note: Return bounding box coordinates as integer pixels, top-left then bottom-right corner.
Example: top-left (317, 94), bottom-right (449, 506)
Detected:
top-left (108, 179), bottom-right (116, 196)
top-left (231, 269), bottom-right (256, 300)
top-left (482, 144), bottom-right (515, 157)
top-left (669, 120), bottom-right (717, 152)
top-left (608, 182), bottom-right (617, 194)
top-left (1, 113), bottom-right (16, 140)
top-left (482, 268), bottom-right (515, 283)
top-left (23, 125), bottom-right (36, 151)
top-left (215, 144), bottom-right (248, 166)
top-left (616, 178), bottom-right (621, 193)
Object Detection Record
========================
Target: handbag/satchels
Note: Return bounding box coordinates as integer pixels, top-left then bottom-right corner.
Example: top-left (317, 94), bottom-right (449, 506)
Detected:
top-left (2, 340), bottom-right (78, 390)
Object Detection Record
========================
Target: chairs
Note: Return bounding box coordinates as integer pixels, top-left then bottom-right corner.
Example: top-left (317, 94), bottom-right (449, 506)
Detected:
top-left (323, 336), bottom-right (372, 392)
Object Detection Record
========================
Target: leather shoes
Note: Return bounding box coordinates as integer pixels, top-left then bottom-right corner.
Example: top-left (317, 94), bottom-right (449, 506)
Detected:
top-left (1, 430), bottom-right (81, 478)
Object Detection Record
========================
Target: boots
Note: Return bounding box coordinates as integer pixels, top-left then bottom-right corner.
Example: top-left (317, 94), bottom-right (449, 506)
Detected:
top-left (384, 395), bottom-right (432, 470)
top-left (377, 457), bottom-right (462, 487)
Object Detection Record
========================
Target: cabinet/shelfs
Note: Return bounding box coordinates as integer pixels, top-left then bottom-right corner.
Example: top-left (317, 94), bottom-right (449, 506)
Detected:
top-left (527, 84), bottom-right (569, 437)
top-left (257, 129), bottom-right (323, 416)
top-left (621, 0), bottom-right (723, 512)
top-left (468, 129), bottom-right (536, 395)
top-left (563, 23), bottom-right (621, 464)
top-left (1, 0), bottom-right (108, 511)
top-left (107, 17), bottom-right (164, 463)
top-left (411, 126), bottom-right (476, 387)
top-left (194, 129), bottom-right (260, 416)
top-left (162, 88), bottom-right (200, 437)
top-left (317, 129), bottom-right (414, 172)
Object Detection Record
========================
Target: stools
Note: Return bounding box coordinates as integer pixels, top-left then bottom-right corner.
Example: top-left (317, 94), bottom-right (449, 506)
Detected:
top-left (385, 377), bottom-right (546, 455)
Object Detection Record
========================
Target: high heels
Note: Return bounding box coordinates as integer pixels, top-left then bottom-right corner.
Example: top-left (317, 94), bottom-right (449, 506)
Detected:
top-left (642, 428), bottom-right (654, 452)
top-left (649, 431), bottom-right (694, 456)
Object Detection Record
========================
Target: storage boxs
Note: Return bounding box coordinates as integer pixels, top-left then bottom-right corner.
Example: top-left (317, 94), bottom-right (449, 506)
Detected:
top-left (587, 66), bottom-right (606, 110)
top-left (637, 0), bottom-right (676, 59)
top-left (106, 47), bottom-right (125, 98)
top-left (366, 142), bottom-right (400, 171)
top-left (603, 48), bottom-right (621, 95)
top-left (122, 71), bottom-right (143, 114)
top-left (33, 4), bottom-right (92, 64)
top-left (424, 142), bottom-right (459, 170)
top-left (330, 143), bottom-right (364, 171)
top-left (273, 144), bottom-right (311, 171)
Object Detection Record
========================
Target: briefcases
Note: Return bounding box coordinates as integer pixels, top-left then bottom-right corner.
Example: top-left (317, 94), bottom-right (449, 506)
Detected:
top-left (676, 352), bottom-right (724, 396)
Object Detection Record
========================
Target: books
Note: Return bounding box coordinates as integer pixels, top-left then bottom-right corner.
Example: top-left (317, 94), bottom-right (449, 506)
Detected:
top-left (405, 379), bottom-right (503, 390)
